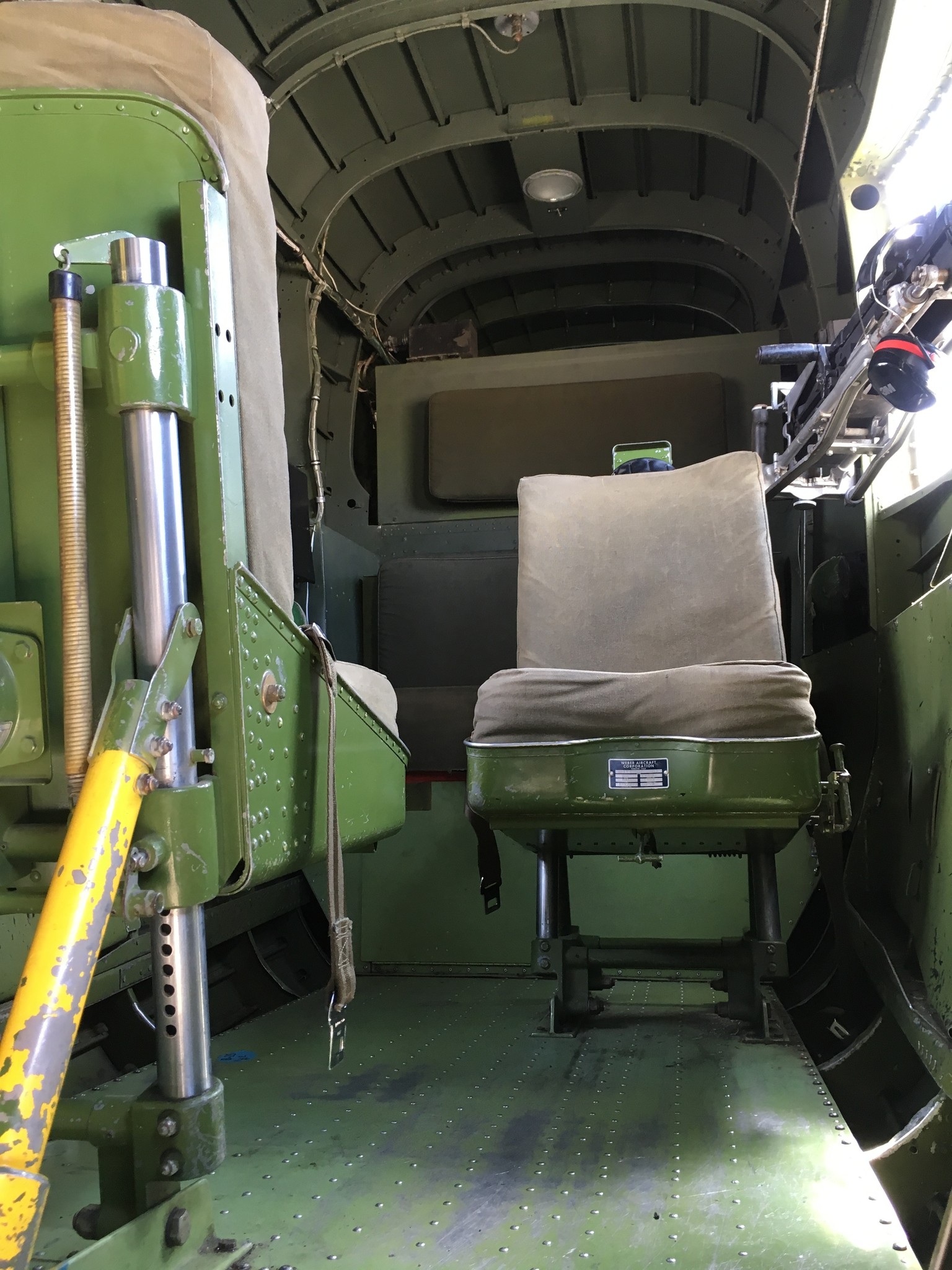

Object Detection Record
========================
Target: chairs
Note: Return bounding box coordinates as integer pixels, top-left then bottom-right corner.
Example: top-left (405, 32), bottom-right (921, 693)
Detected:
top-left (461, 450), bottom-right (854, 1044)
top-left (1, 1), bottom-right (408, 1270)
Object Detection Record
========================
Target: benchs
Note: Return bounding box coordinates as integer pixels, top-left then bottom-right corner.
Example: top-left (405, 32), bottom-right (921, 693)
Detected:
top-left (375, 545), bottom-right (791, 774)
